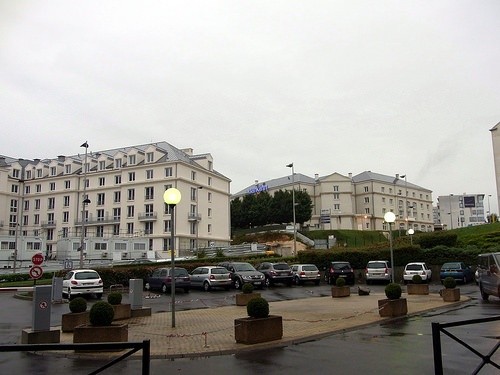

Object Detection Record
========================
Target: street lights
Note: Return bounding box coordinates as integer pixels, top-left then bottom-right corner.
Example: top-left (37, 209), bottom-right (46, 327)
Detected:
top-left (163, 187), bottom-right (181, 328)
top-left (80, 141), bottom-right (91, 268)
top-left (400, 175), bottom-right (410, 244)
top-left (408, 228), bottom-right (414, 245)
top-left (384, 212), bottom-right (397, 283)
top-left (488, 195), bottom-right (492, 224)
top-left (286, 162), bottom-right (296, 256)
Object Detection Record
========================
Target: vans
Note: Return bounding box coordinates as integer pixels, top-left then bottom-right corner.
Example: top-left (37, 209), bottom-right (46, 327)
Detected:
top-left (474, 252), bottom-right (500, 301)
top-left (365, 260), bottom-right (392, 284)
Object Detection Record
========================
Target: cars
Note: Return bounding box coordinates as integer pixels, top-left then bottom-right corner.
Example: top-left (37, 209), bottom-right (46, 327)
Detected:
top-left (61, 269), bottom-right (103, 301)
top-left (403, 262), bottom-right (432, 285)
top-left (189, 266), bottom-right (232, 292)
top-left (144, 267), bottom-right (190, 294)
top-left (289, 264), bottom-right (321, 286)
top-left (439, 261), bottom-right (473, 285)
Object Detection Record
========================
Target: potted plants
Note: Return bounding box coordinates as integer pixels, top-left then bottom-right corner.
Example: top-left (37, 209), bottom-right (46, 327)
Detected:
top-left (107, 291), bottom-right (131, 322)
top-left (234, 296), bottom-right (283, 344)
top-left (72, 300), bottom-right (129, 352)
top-left (61, 298), bottom-right (90, 332)
top-left (378, 283), bottom-right (408, 317)
top-left (235, 282), bottom-right (262, 306)
top-left (332, 278), bottom-right (350, 298)
top-left (440, 277), bottom-right (460, 302)
top-left (407, 275), bottom-right (429, 295)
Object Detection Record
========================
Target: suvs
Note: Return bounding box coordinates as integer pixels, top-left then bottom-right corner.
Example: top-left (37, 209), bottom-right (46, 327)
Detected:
top-left (256, 261), bottom-right (294, 287)
top-left (323, 261), bottom-right (355, 285)
top-left (218, 262), bottom-right (266, 290)
